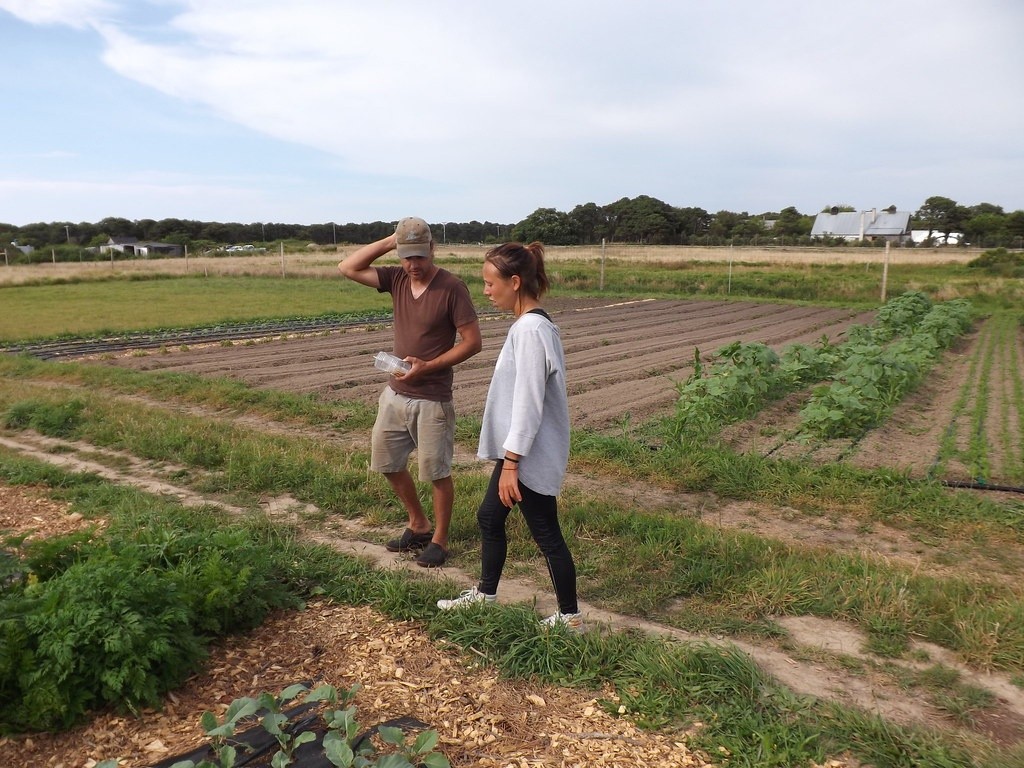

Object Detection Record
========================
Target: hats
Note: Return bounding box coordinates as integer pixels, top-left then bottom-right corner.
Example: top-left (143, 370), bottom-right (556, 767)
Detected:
top-left (396, 217), bottom-right (432, 258)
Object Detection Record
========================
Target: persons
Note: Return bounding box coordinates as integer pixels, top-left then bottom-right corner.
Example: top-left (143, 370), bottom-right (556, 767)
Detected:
top-left (337, 217), bottom-right (483, 567)
top-left (436, 241), bottom-right (582, 628)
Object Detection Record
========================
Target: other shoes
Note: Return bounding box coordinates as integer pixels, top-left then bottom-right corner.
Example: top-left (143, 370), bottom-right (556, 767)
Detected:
top-left (416, 542), bottom-right (449, 567)
top-left (386, 528), bottom-right (433, 552)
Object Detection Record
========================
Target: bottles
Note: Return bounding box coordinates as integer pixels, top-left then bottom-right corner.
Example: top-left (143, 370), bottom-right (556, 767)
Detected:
top-left (373, 351), bottom-right (412, 378)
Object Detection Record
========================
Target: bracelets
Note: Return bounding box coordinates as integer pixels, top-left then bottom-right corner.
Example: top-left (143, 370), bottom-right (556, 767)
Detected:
top-left (504, 456), bottom-right (518, 463)
top-left (502, 467), bottom-right (519, 470)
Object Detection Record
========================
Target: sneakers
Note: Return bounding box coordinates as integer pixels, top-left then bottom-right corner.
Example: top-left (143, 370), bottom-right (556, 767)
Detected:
top-left (539, 609), bottom-right (583, 631)
top-left (437, 586), bottom-right (497, 611)
